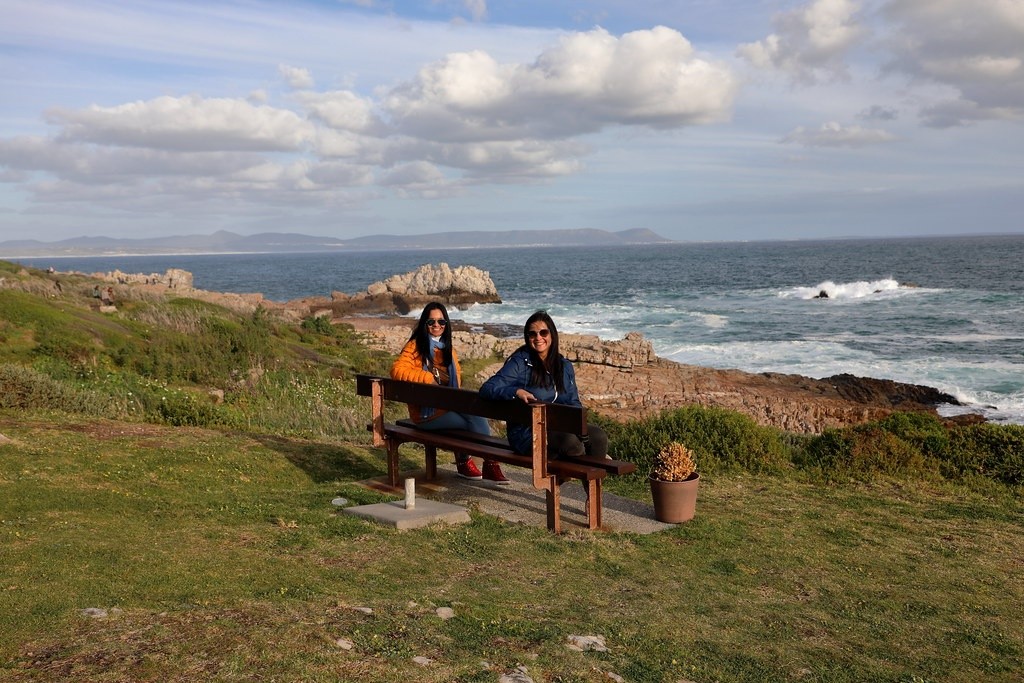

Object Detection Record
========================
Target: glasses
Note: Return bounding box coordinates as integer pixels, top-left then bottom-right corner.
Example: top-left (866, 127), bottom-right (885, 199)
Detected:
top-left (426, 319), bottom-right (447, 325)
top-left (527, 329), bottom-right (550, 337)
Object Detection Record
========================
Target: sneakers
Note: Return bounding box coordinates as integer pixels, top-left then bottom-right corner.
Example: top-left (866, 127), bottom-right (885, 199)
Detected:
top-left (482, 462), bottom-right (510, 484)
top-left (457, 458), bottom-right (482, 480)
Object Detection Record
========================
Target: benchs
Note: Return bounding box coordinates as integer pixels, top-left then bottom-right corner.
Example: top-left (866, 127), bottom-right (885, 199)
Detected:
top-left (355, 373), bottom-right (634, 531)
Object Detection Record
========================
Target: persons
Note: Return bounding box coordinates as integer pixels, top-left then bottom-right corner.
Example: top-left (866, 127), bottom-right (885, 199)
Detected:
top-left (390, 302), bottom-right (512, 485)
top-left (477, 312), bottom-right (613, 483)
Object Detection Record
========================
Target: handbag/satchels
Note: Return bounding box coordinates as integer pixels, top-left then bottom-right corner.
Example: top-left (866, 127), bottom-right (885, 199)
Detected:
top-left (429, 362), bottom-right (450, 386)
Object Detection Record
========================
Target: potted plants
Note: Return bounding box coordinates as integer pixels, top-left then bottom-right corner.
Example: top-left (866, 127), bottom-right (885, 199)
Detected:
top-left (647, 442), bottom-right (700, 523)
